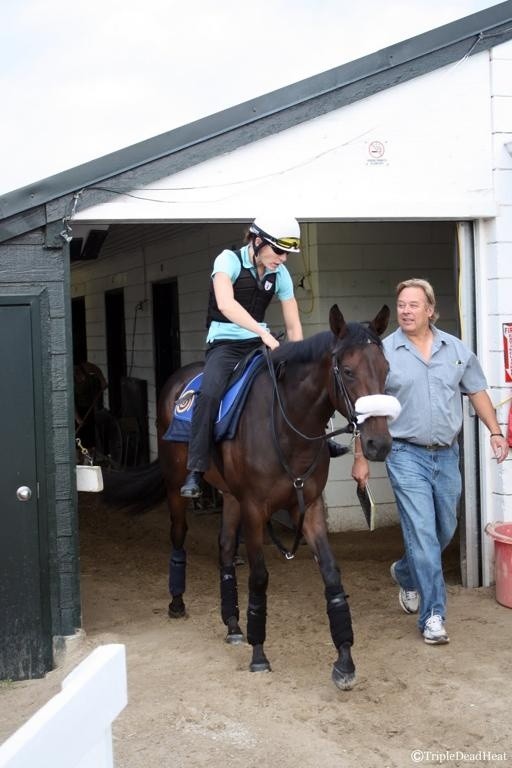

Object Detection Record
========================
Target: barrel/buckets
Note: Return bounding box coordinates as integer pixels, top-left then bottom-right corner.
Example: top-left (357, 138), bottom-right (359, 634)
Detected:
top-left (485, 518), bottom-right (512, 611)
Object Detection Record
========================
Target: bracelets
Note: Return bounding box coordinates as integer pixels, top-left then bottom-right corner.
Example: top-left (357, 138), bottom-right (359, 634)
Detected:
top-left (488, 433), bottom-right (505, 437)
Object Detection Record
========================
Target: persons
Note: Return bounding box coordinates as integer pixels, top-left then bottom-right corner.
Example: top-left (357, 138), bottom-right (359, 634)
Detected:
top-left (179, 204), bottom-right (351, 497)
top-left (350, 277), bottom-right (509, 643)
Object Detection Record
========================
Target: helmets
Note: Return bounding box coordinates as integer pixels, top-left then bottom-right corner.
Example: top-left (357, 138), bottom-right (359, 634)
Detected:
top-left (249, 211), bottom-right (302, 254)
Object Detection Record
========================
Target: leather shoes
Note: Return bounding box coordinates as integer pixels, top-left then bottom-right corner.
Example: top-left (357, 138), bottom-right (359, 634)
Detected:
top-left (326, 437), bottom-right (350, 458)
top-left (179, 470), bottom-right (206, 498)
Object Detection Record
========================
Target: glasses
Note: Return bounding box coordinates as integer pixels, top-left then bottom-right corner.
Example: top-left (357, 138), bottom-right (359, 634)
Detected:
top-left (264, 241), bottom-right (291, 256)
top-left (274, 235), bottom-right (302, 250)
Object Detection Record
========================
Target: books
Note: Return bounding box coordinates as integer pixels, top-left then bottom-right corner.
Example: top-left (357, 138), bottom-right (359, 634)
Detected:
top-left (356, 483), bottom-right (375, 532)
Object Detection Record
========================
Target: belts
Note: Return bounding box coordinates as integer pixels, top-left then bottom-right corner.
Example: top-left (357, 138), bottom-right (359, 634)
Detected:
top-left (392, 436), bottom-right (456, 450)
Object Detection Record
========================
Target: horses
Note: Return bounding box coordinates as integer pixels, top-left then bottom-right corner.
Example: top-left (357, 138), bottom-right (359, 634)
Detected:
top-left (104, 304), bottom-right (393, 690)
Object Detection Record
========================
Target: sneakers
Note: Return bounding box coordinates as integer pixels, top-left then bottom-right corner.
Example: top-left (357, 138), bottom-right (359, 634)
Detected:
top-left (389, 559), bottom-right (421, 615)
top-left (420, 613), bottom-right (451, 646)
